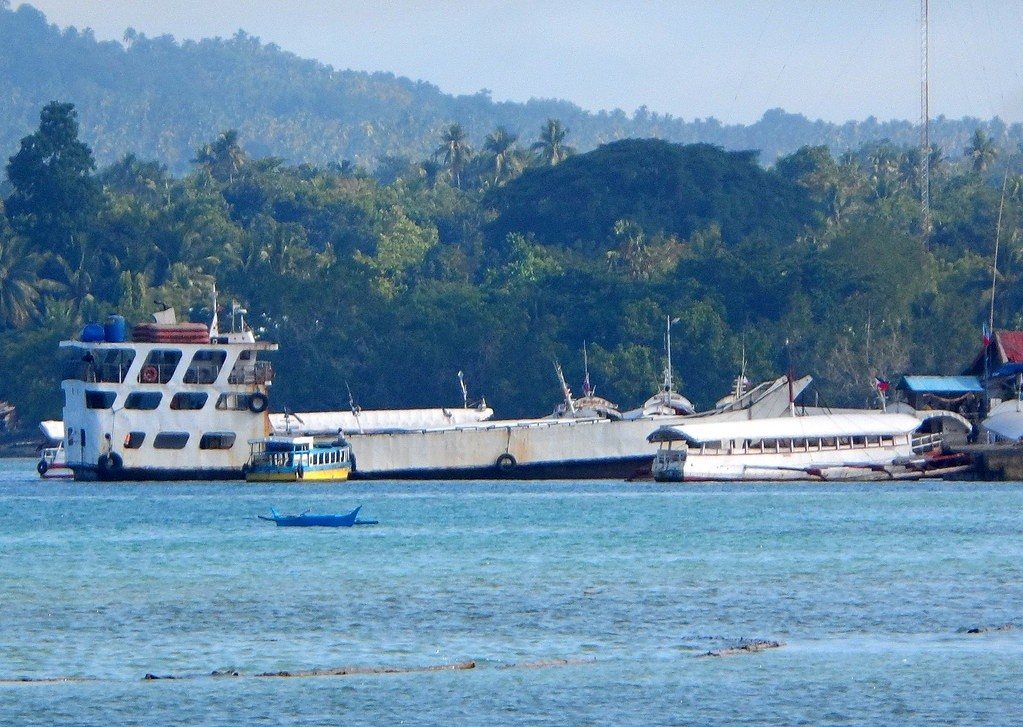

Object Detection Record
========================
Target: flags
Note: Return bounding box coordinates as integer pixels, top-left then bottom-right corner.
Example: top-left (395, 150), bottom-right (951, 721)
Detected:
top-left (874, 377), bottom-right (891, 392)
top-left (983, 323), bottom-right (989, 345)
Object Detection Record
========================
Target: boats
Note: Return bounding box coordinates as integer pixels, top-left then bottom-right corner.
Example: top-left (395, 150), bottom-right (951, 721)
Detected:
top-left (950, 409), bottom-right (1022, 483)
top-left (243, 406), bottom-right (357, 485)
top-left (132, 322), bottom-right (211, 343)
top-left (645, 337), bottom-right (977, 483)
top-left (36, 420), bottom-right (74, 479)
top-left (256, 503), bottom-right (380, 527)
top-left (57, 281), bottom-right (813, 483)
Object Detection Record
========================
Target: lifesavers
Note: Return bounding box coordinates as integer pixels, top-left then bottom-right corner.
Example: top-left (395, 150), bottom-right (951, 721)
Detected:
top-left (99, 452), bottom-right (123, 475)
top-left (497, 453), bottom-right (516, 473)
top-left (248, 392), bottom-right (268, 413)
top-left (242, 464), bottom-right (249, 474)
top-left (298, 464), bottom-right (303, 477)
top-left (143, 367), bottom-right (158, 382)
top-left (36, 460), bottom-right (48, 474)
